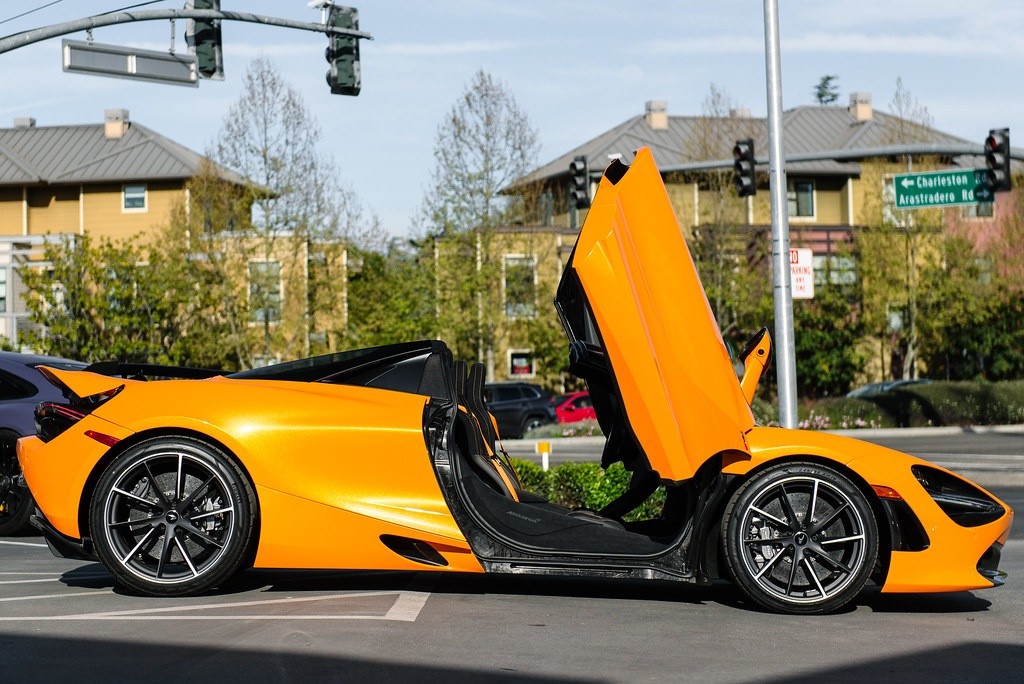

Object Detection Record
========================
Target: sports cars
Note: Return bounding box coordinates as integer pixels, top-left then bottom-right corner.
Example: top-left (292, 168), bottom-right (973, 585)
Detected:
top-left (12, 144), bottom-right (1016, 614)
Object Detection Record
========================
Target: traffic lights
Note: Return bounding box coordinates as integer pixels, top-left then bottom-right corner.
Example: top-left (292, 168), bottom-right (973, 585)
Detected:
top-left (732, 138), bottom-right (756, 196)
top-left (983, 128), bottom-right (1011, 192)
top-left (567, 155), bottom-right (591, 209)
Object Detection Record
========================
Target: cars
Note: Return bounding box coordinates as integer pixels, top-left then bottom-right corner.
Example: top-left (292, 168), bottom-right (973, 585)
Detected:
top-left (848, 376), bottom-right (932, 397)
top-left (0, 349), bottom-right (132, 538)
top-left (555, 391), bottom-right (597, 425)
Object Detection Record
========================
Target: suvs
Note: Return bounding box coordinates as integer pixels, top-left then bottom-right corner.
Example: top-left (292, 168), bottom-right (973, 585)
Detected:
top-left (481, 382), bottom-right (553, 440)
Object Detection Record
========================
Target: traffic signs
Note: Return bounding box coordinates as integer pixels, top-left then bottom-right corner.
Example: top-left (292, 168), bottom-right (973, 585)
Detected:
top-left (892, 169), bottom-right (993, 210)
